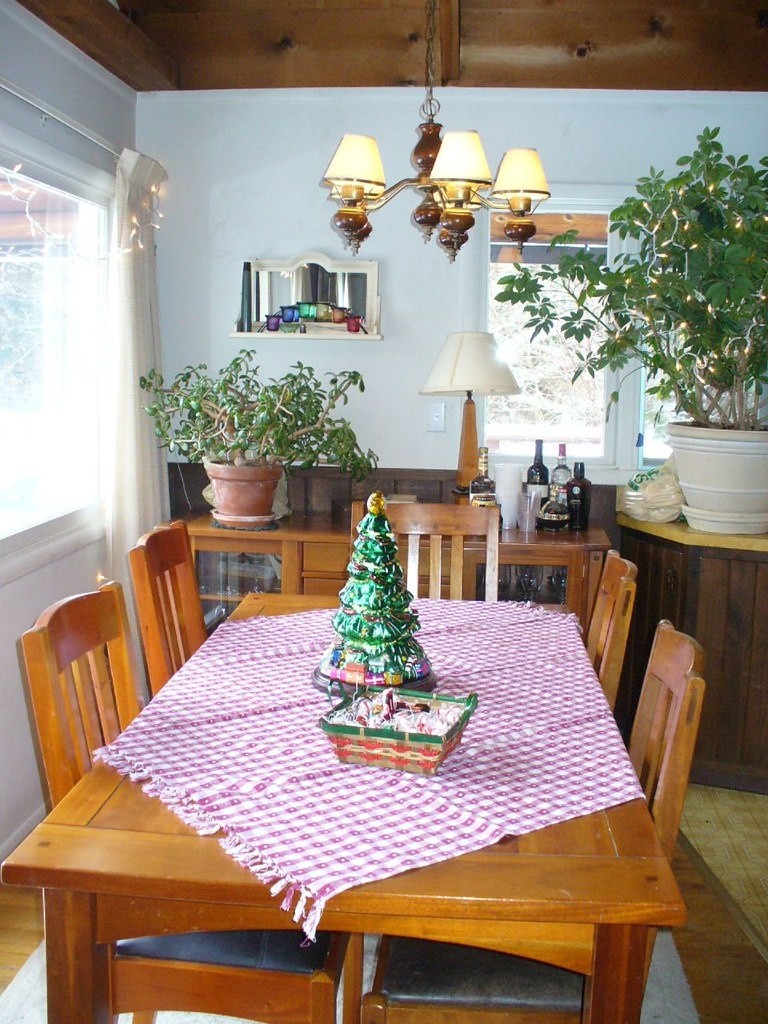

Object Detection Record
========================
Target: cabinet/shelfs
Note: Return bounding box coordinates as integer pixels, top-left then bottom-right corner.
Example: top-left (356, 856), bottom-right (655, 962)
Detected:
top-left (153, 509), bottom-right (612, 626)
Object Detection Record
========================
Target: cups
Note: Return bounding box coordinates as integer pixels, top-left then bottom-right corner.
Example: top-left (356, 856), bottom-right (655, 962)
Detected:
top-left (265, 302), bottom-right (364, 332)
top-left (493, 463), bottom-right (542, 533)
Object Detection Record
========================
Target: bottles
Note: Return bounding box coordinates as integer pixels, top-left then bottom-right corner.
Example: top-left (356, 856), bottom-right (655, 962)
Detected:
top-left (567, 462), bottom-right (591, 531)
top-left (468, 447), bottom-right (496, 507)
top-left (535, 484), bottom-right (572, 533)
top-left (496, 504), bottom-right (503, 534)
top-left (551, 443), bottom-right (572, 508)
top-left (527, 439), bottom-right (549, 530)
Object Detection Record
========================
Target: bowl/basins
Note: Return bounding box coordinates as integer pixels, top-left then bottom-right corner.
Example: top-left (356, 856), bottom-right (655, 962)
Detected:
top-left (278, 323), bottom-right (300, 332)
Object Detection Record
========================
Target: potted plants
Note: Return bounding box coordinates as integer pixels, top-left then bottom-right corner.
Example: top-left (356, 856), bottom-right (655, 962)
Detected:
top-left (499, 128), bottom-right (768, 536)
top-left (132, 355), bottom-right (382, 531)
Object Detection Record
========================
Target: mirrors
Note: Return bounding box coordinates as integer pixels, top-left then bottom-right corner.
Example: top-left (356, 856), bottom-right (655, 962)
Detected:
top-left (237, 250), bottom-right (383, 338)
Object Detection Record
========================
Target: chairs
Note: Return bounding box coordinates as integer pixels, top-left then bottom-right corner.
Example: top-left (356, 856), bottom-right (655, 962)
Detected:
top-left (15, 502), bottom-right (705, 1024)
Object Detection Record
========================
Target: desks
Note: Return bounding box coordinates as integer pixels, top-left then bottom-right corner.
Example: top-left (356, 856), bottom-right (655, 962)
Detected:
top-left (1, 589), bottom-right (687, 1023)
top-left (605, 508), bottom-right (768, 798)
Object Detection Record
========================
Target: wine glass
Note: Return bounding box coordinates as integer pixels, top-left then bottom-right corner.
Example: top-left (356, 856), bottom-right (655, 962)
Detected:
top-left (498, 563), bottom-right (567, 605)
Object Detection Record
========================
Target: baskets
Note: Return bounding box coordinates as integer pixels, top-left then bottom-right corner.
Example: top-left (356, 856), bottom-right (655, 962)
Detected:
top-left (321, 676), bottom-right (478, 775)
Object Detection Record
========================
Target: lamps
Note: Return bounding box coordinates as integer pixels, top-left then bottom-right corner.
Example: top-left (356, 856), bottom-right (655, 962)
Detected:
top-left (421, 332), bottom-right (521, 494)
top-left (322, 1), bottom-right (549, 264)
